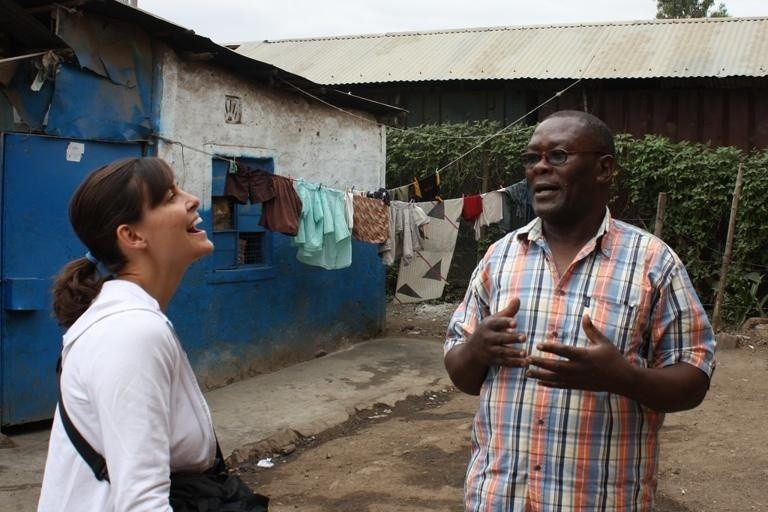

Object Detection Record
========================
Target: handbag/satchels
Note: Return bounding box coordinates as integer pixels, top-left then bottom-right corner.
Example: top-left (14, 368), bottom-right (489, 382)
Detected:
top-left (168, 458), bottom-right (269, 512)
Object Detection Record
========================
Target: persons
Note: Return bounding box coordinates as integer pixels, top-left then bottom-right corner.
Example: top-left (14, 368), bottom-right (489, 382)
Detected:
top-left (443, 111), bottom-right (716, 512)
top-left (37, 157), bottom-right (217, 512)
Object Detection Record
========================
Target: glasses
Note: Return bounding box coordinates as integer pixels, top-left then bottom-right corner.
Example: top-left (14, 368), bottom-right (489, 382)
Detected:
top-left (520, 148), bottom-right (601, 169)
